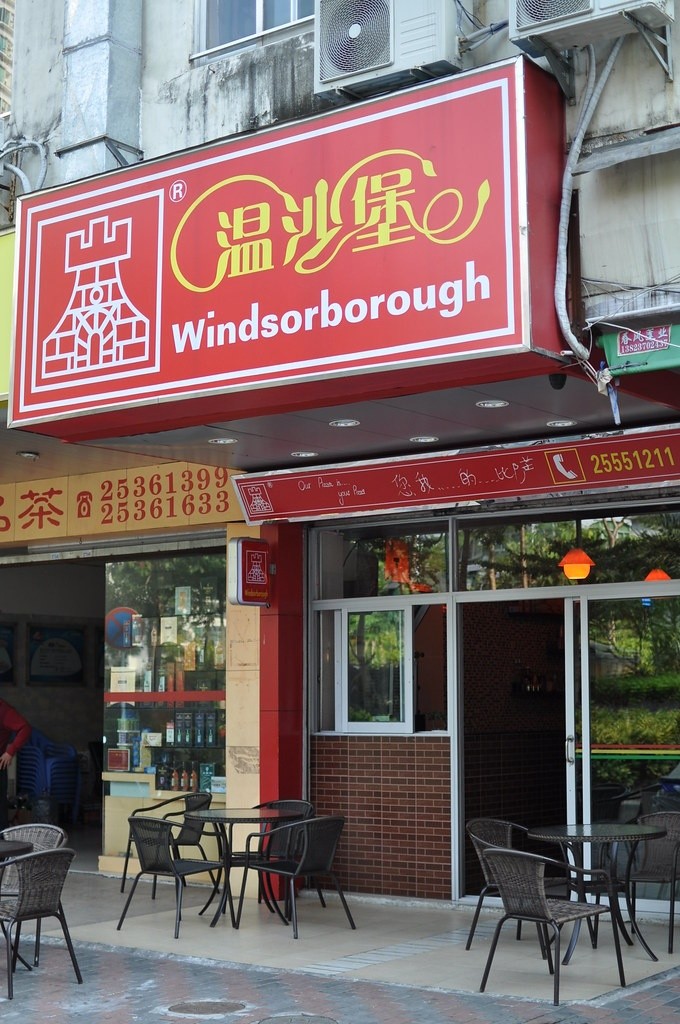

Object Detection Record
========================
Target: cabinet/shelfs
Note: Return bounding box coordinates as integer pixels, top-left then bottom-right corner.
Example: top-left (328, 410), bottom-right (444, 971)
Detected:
top-left (104, 611), bottom-right (226, 749)
top-left (508, 610), bottom-right (563, 699)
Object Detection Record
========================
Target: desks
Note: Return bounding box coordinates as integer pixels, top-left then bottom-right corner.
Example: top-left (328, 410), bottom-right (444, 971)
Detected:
top-left (0, 839), bottom-right (33, 971)
top-left (184, 808), bottom-right (304, 927)
top-left (526, 823), bottom-right (667, 966)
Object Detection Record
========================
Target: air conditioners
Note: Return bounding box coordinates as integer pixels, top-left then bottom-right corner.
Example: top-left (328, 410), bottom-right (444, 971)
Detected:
top-left (508, 0), bottom-right (674, 59)
top-left (313, 0), bottom-right (473, 106)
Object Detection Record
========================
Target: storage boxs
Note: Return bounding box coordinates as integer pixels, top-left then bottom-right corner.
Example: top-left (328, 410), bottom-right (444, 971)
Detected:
top-left (175, 586), bottom-right (192, 615)
top-left (122, 619), bottom-right (132, 648)
top-left (132, 613), bottom-right (143, 647)
top-left (194, 713), bottom-right (205, 748)
top-left (211, 776), bottom-right (226, 794)
top-left (199, 762), bottom-right (218, 793)
top-left (174, 713), bottom-right (184, 747)
top-left (166, 662), bottom-right (175, 691)
top-left (183, 641), bottom-right (197, 672)
top-left (140, 656), bottom-right (155, 692)
top-left (205, 712), bottom-right (217, 748)
top-left (155, 665), bottom-right (166, 692)
top-left (175, 661), bottom-right (186, 692)
top-left (184, 713), bottom-right (194, 748)
top-left (110, 667), bottom-right (136, 692)
top-left (160, 616), bottom-right (186, 646)
top-left (216, 708), bottom-right (226, 748)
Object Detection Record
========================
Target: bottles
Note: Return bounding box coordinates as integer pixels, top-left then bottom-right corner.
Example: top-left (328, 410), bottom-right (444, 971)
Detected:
top-left (156, 758), bottom-right (167, 790)
top-left (190, 761), bottom-right (199, 791)
top-left (170, 760), bottom-right (180, 792)
top-left (208, 729), bottom-right (213, 743)
top-left (180, 762), bottom-right (190, 792)
top-left (197, 729), bottom-right (201, 743)
top-left (212, 631), bottom-right (225, 670)
top-left (185, 729), bottom-right (190, 744)
top-left (177, 728), bottom-right (182, 743)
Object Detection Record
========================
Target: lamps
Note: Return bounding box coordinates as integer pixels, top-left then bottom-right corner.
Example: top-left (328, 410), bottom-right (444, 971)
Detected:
top-left (644, 569), bottom-right (674, 599)
top-left (558, 548), bottom-right (596, 580)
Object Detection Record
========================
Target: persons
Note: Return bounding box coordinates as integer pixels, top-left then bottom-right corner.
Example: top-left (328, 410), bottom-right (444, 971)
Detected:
top-left (1, 694), bottom-right (31, 833)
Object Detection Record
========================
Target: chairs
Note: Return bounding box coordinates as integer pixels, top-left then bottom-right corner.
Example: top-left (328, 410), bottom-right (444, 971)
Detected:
top-left (465, 818), bottom-right (577, 951)
top-left (222, 799), bottom-right (326, 919)
top-left (117, 816), bottom-right (236, 939)
top-left (235, 815), bottom-right (356, 939)
top-left (479, 848), bottom-right (626, 1006)
top-left (11, 726), bottom-right (82, 827)
top-left (0, 823), bottom-right (69, 973)
top-left (120, 792), bottom-right (220, 900)
top-left (577, 783), bottom-right (631, 879)
top-left (0, 848), bottom-right (83, 1000)
top-left (594, 811), bottom-right (680, 954)
top-left (596, 783), bottom-right (663, 880)
